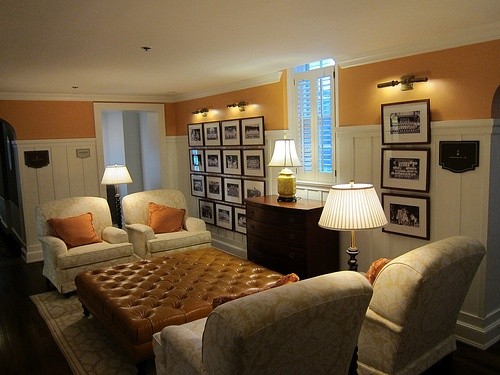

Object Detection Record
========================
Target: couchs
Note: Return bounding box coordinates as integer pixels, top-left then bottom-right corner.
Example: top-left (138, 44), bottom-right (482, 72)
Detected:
top-left (151, 234), bottom-right (486, 375)
top-left (34, 188), bottom-right (213, 300)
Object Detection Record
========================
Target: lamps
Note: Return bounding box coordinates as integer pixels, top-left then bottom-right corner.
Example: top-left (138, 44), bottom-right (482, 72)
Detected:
top-left (267, 139), bottom-right (303, 202)
top-left (101, 164), bottom-right (133, 229)
top-left (377, 74), bottom-right (428, 91)
top-left (319, 180), bottom-right (389, 270)
top-left (192, 108), bottom-right (208, 117)
top-left (227, 101), bottom-right (248, 111)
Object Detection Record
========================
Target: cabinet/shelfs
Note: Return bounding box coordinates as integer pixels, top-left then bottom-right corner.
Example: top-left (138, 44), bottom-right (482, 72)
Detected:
top-left (243, 194), bottom-right (340, 280)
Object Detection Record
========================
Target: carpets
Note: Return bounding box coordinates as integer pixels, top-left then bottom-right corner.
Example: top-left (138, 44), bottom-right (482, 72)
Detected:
top-left (30, 291), bottom-right (139, 375)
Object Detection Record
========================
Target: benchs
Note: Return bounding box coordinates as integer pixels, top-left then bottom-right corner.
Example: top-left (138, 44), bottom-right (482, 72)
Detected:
top-left (74, 247), bottom-right (284, 375)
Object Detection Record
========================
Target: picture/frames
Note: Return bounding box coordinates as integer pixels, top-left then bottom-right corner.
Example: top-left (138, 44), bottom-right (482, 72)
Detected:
top-left (188, 116), bottom-right (266, 236)
top-left (382, 192), bottom-right (430, 240)
top-left (381, 98), bottom-right (431, 146)
top-left (380, 147), bottom-right (431, 193)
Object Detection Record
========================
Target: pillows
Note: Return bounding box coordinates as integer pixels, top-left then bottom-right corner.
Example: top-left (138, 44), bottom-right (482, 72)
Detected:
top-left (365, 258), bottom-right (390, 285)
top-left (47, 211), bottom-right (103, 250)
top-left (211, 272), bottom-right (299, 309)
top-left (148, 201), bottom-right (186, 234)
